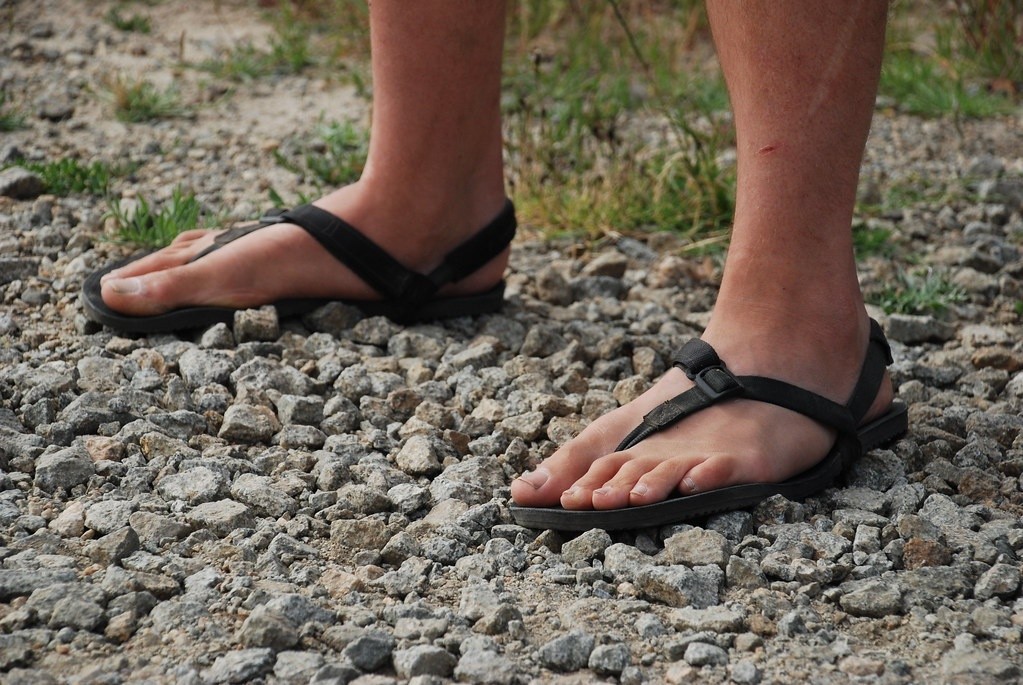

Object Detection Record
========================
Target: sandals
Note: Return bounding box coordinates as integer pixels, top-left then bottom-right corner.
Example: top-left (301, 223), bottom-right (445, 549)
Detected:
top-left (508, 318), bottom-right (910, 532)
top-left (78, 198), bottom-right (518, 336)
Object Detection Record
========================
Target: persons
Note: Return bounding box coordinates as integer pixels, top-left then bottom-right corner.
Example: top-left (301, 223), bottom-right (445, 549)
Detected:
top-left (82, 0), bottom-right (909, 539)
